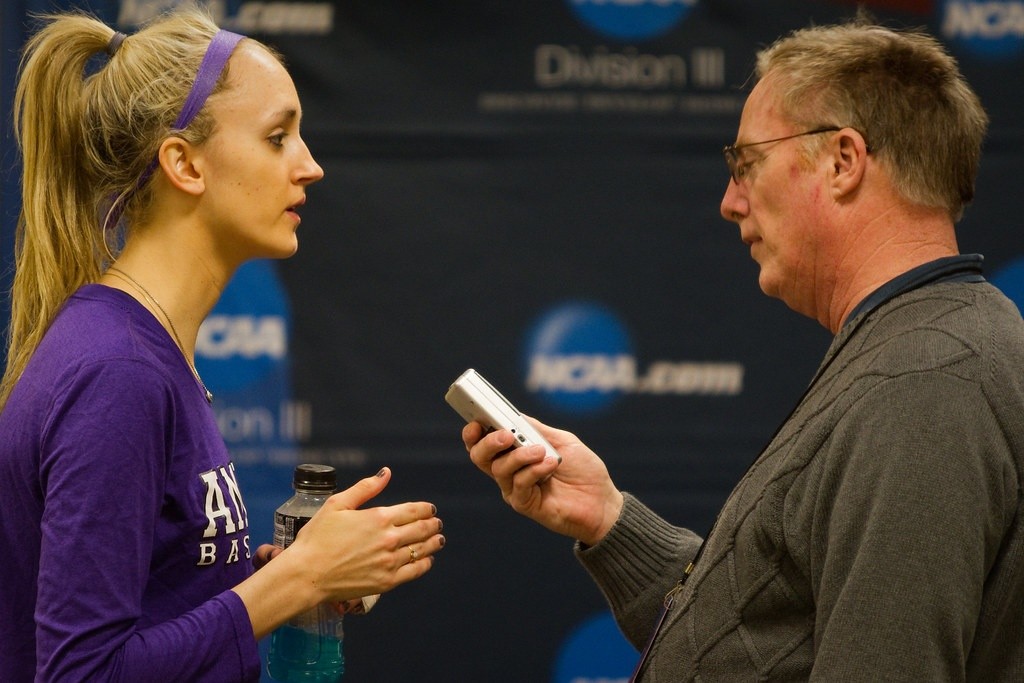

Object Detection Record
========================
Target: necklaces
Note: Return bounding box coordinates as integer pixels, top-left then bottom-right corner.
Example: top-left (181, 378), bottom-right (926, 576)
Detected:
top-left (108, 264), bottom-right (213, 406)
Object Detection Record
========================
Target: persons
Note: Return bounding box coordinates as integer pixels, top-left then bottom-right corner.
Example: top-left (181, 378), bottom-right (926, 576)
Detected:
top-left (0, 0), bottom-right (446, 683)
top-left (461, 25), bottom-right (1024, 683)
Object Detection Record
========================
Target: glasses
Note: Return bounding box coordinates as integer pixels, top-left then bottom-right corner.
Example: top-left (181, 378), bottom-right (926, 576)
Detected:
top-left (722, 126), bottom-right (871, 185)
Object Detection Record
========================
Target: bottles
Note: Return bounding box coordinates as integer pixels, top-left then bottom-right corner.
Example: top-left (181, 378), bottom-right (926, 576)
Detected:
top-left (268, 464), bottom-right (344, 683)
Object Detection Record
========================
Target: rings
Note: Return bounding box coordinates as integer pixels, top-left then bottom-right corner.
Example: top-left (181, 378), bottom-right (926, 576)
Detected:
top-left (406, 544), bottom-right (417, 564)
